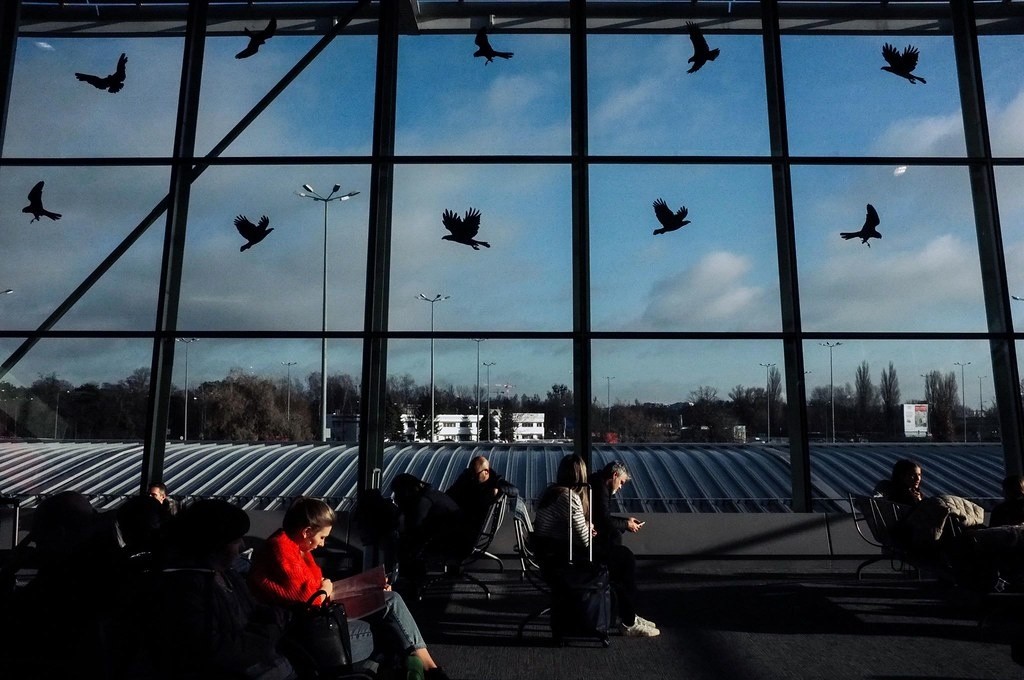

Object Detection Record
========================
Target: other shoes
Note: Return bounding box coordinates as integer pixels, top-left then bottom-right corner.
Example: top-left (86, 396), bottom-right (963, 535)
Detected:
top-left (423, 666), bottom-right (449, 680)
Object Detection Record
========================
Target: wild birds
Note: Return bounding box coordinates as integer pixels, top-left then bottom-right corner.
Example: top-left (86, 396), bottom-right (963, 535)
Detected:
top-left (686, 21), bottom-right (720, 73)
top-left (75, 52), bottom-right (128, 93)
top-left (881, 42), bottom-right (926, 84)
top-left (652, 198), bottom-right (691, 236)
top-left (234, 214), bottom-right (274, 251)
top-left (474, 27), bottom-right (514, 66)
top-left (441, 207), bottom-right (490, 250)
top-left (22, 181), bottom-right (62, 224)
top-left (234, 18), bottom-right (277, 59)
top-left (840, 203), bottom-right (882, 248)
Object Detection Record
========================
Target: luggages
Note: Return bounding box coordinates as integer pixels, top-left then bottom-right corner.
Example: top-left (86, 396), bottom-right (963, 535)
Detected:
top-left (551, 483), bottom-right (611, 646)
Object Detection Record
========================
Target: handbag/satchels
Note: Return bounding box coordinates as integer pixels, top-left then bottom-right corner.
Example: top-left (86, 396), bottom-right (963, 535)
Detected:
top-left (283, 590), bottom-right (353, 675)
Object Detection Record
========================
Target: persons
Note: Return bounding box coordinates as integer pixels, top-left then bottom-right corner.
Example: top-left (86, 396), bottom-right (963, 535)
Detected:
top-left (0, 456), bottom-right (520, 680)
top-left (533, 453), bottom-right (660, 637)
top-left (872, 459), bottom-right (1024, 667)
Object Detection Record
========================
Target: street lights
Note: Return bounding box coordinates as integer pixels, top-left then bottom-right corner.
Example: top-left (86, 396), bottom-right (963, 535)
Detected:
top-left (175, 338), bottom-right (200, 440)
top-left (281, 361), bottom-right (297, 422)
top-left (819, 342), bottom-right (843, 443)
top-left (414, 293), bottom-right (451, 443)
top-left (293, 183), bottom-right (361, 442)
top-left (954, 362), bottom-right (971, 443)
top-left (760, 363), bottom-right (776, 443)
top-left (54, 388), bottom-right (70, 440)
top-left (978, 376), bottom-right (987, 417)
top-left (470, 338), bottom-right (487, 442)
top-left (602, 376), bottom-right (615, 433)
top-left (483, 362), bottom-right (496, 442)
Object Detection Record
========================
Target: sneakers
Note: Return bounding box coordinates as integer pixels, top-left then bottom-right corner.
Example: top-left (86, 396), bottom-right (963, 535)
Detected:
top-left (621, 614), bottom-right (660, 637)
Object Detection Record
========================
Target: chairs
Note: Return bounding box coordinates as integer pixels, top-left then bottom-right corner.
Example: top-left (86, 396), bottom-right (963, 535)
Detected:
top-left (416, 494), bottom-right (611, 648)
top-left (847, 491), bottom-right (922, 584)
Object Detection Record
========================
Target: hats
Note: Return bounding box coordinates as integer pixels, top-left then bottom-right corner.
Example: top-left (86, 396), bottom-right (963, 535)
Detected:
top-left (169, 500), bottom-right (250, 550)
